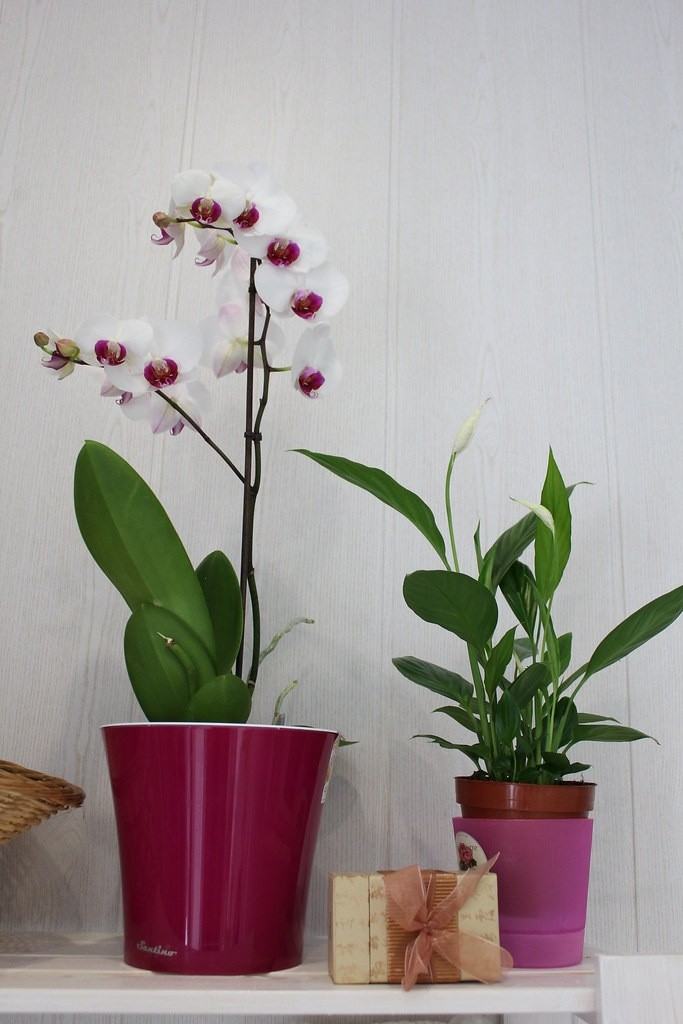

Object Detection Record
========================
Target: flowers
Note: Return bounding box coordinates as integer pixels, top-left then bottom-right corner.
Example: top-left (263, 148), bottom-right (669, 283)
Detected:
top-left (283, 396), bottom-right (683, 785)
top-left (33, 166), bottom-right (362, 749)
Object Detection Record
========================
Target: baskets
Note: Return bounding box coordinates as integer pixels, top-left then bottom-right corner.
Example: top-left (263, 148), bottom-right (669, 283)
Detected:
top-left (0, 760), bottom-right (86, 845)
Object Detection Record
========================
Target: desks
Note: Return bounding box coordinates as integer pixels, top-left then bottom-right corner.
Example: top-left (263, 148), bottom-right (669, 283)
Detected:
top-left (0, 931), bottom-right (597, 1024)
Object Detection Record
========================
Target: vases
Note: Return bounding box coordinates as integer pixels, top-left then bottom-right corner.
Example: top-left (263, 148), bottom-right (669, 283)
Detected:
top-left (99, 722), bottom-right (339, 975)
top-left (452, 817), bottom-right (593, 970)
top-left (454, 776), bottom-right (597, 819)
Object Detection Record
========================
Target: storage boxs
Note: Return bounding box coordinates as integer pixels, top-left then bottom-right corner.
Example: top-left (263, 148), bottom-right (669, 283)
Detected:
top-left (327, 871), bottom-right (502, 986)
